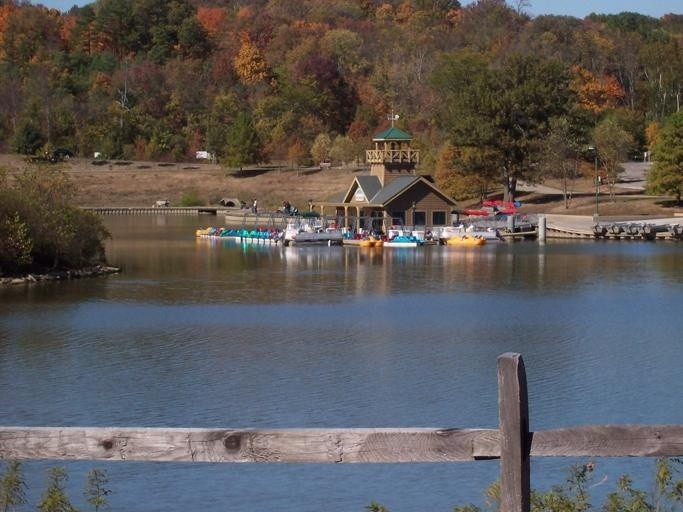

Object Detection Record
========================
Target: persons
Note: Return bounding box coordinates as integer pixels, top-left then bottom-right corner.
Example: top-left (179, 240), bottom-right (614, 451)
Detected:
top-left (283, 200), bottom-right (290, 215)
top-left (425, 229), bottom-right (433, 241)
top-left (240, 198), bottom-right (257, 213)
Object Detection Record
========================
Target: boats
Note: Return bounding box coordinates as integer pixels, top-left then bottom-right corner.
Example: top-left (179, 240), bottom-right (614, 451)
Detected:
top-left (446, 234), bottom-right (487, 245)
top-left (359, 234), bottom-right (383, 248)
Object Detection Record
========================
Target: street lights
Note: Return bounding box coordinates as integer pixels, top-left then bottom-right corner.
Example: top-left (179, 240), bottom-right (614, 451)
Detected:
top-left (588, 146), bottom-right (599, 213)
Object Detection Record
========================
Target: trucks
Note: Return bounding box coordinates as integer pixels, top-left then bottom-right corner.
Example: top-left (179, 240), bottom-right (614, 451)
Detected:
top-left (194, 150), bottom-right (214, 161)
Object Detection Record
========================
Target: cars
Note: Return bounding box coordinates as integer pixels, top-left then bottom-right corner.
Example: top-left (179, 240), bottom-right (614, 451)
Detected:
top-left (45, 147), bottom-right (74, 158)
